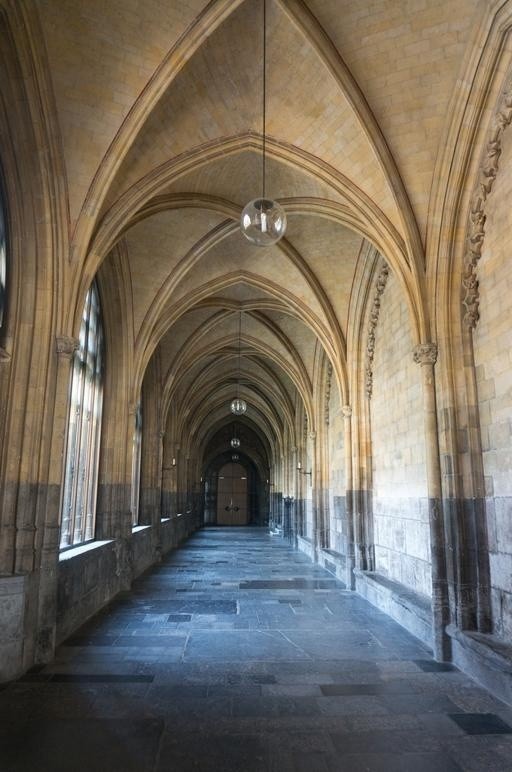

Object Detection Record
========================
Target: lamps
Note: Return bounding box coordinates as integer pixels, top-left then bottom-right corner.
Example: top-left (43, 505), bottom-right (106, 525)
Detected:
top-left (240, 2), bottom-right (288, 246)
top-left (230, 312), bottom-right (246, 415)
top-left (231, 438), bottom-right (240, 448)
top-left (232, 453), bottom-right (239, 462)
